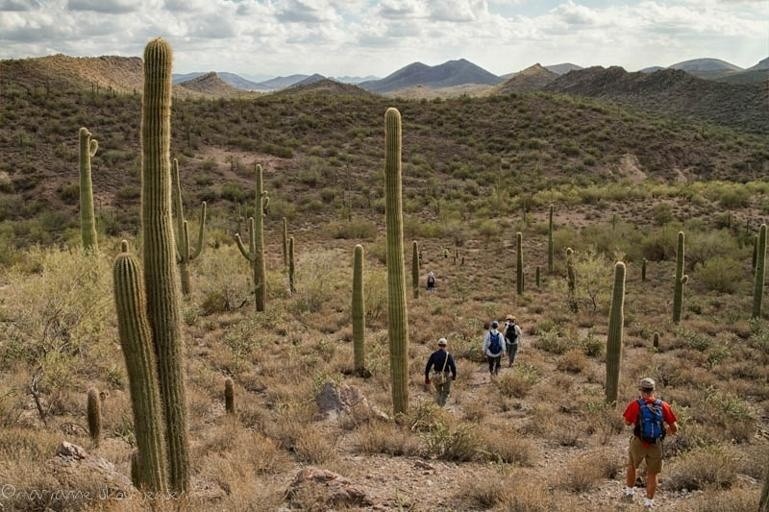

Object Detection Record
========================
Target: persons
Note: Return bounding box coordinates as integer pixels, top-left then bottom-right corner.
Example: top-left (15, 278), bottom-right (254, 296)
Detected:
top-left (503, 315), bottom-right (523, 368)
top-left (621, 377), bottom-right (679, 509)
top-left (425, 337), bottom-right (457, 410)
top-left (503, 314), bottom-right (512, 355)
top-left (426, 271), bottom-right (437, 290)
top-left (481, 321), bottom-right (507, 377)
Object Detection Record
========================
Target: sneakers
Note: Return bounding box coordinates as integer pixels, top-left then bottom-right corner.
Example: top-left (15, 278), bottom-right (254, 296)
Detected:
top-left (509, 361), bottom-right (513, 366)
top-left (618, 494), bottom-right (655, 512)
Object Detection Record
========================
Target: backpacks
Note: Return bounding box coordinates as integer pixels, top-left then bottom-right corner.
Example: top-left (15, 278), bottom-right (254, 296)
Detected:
top-left (505, 325), bottom-right (518, 343)
top-left (634, 399), bottom-right (666, 445)
top-left (489, 331), bottom-right (501, 354)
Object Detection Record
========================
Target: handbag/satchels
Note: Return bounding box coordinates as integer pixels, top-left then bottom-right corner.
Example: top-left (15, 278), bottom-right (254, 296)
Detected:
top-left (433, 372), bottom-right (445, 385)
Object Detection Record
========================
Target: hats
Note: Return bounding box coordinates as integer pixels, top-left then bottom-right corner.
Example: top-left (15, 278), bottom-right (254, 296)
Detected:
top-left (491, 321), bottom-right (500, 327)
top-left (641, 378), bottom-right (657, 393)
top-left (505, 314), bottom-right (516, 319)
top-left (438, 338), bottom-right (447, 345)
